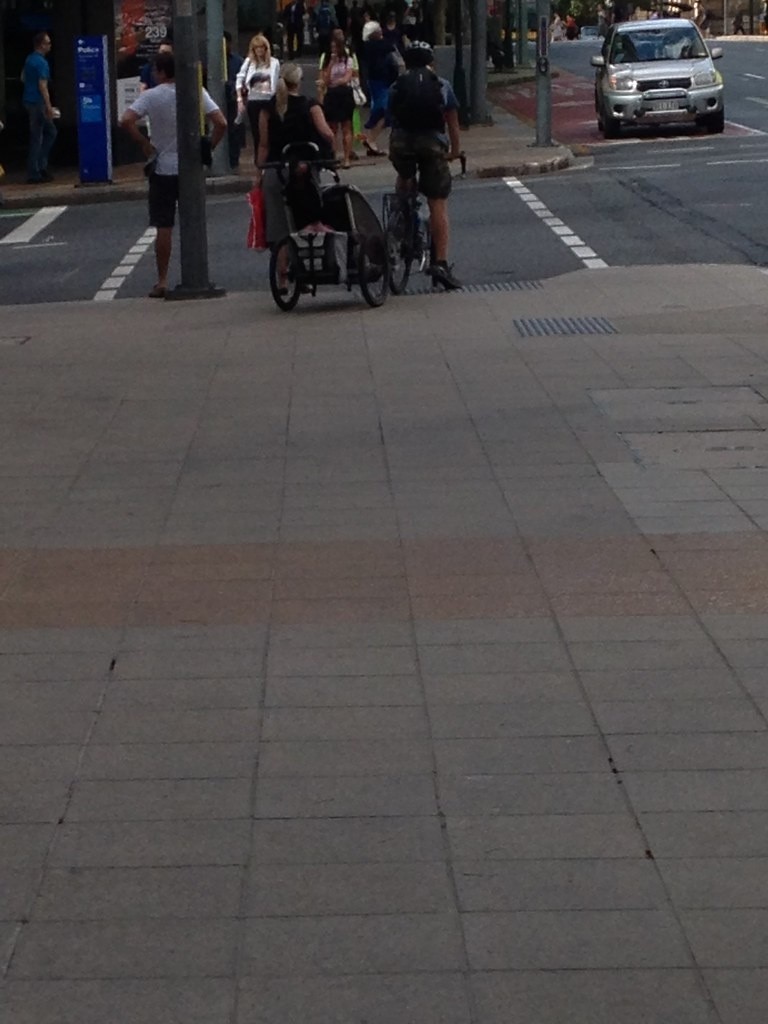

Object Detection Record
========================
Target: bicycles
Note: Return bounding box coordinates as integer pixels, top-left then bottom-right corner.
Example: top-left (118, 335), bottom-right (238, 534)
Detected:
top-left (366, 147), bottom-right (468, 295)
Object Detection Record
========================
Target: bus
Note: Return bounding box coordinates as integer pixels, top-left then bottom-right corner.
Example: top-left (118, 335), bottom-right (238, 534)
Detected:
top-left (501, 9), bottom-right (537, 42)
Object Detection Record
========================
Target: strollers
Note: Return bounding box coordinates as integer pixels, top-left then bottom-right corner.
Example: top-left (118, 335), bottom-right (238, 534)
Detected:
top-left (258, 156), bottom-right (392, 312)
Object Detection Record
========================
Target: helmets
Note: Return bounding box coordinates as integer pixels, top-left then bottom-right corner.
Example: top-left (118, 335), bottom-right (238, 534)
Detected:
top-left (402, 40), bottom-right (435, 62)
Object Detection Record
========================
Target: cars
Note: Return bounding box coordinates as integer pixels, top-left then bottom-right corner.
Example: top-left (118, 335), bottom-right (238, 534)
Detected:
top-left (579, 27), bottom-right (600, 41)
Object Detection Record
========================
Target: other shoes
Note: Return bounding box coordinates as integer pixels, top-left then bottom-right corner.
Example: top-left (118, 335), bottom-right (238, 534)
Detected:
top-left (278, 288), bottom-right (287, 295)
top-left (28, 169), bottom-right (56, 183)
top-left (363, 140), bottom-right (388, 157)
top-left (148, 286), bottom-right (168, 297)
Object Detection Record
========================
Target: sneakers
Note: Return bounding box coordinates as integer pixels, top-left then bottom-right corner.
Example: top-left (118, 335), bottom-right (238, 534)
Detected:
top-left (431, 265), bottom-right (463, 290)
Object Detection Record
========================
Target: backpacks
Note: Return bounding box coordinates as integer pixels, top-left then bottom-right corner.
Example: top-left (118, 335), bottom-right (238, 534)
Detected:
top-left (390, 68), bottom-right (446, 134)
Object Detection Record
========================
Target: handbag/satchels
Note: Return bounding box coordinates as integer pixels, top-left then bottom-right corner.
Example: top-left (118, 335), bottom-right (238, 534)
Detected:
top-left (350, 75), bottom-right (367, 107)
top-left (240, 60), bottom-right (251, 98)
top-left (246, 187), bottom-right (268, 250)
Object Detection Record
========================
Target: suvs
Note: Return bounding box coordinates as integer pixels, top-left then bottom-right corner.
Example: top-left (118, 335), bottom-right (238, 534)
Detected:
top-left (589, 18), bottom-right (724, 140)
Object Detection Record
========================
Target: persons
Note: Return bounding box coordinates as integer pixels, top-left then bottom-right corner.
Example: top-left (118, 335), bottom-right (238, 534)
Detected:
top-left (255, 61), bottom-right (340, 184)
top-left (761, 0), bottom-right (768, 32)
top-left (649, 2), bottom-right (660, 20)
top-left (280, 51), bottom-right (305, 97)
top-left (550, 11), bottom-right (561, 34)
top-left (20, 32), bottom-right (58, 183)
top-left (261, 0), bottom-right (435, 169)
top-left (732, 5), bottom-right (746, 35)
top-left (562, 13), bottom-right (576, 40)
top-left (597, 4), bottom-right (609, 38)
top-left (121, 48), bottom-right (228, 298)
top-left (692, 0), bottom-right (706, 27)
top-left (224, 31), bottom-right (246, 174)
top-left (668, 0), bottom-right (681, 18)
top-left (383, 41), bottom-right (461, 289)
top-left (487, 15), bottom-right (507, 72)
top-left (140, 39), bottom-right (174, 143)
top-left (234, 36), bottom-right (280, 167)
top-left (604, 0), bottom-right (621, 25)
